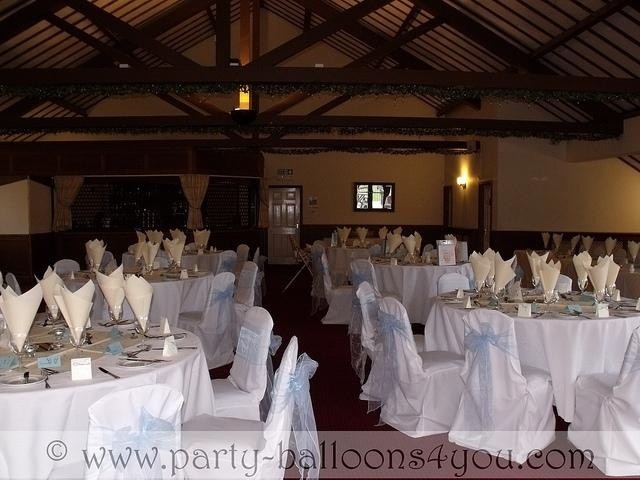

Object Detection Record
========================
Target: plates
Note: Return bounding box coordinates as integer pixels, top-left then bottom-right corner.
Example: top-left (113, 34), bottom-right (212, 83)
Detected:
top-left (0, 320), bottom-right (186, 387)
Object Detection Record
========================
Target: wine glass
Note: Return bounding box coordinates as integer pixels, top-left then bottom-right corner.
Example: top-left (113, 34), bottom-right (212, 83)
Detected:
top-left (339, 236), bottom-right (423, 265)
top-left (545, 243), bottom-right (637, 270)
top-left (87, 243), bottom-right (208, 277)
top-left (473, 276), bottom-right (616, 313)
top-left (10, 304), bottom-right (150, 373)
